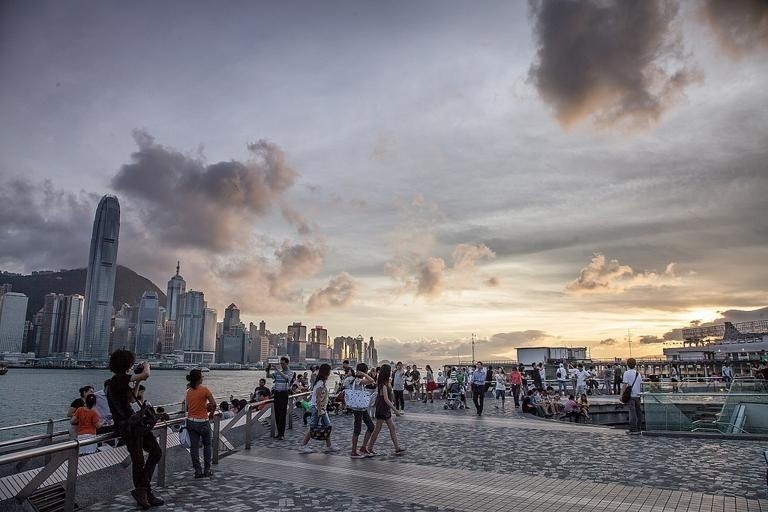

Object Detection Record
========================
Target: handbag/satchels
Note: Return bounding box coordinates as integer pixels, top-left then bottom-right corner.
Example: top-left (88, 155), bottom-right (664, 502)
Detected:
top-left (128, 405), bottom-right (157, 434)
top-left (179, 425), bottom-right (202, 449)
top-left (557, 372), bottom-right (562, 378)
top-left (344, 380), bottom-right (370, 410)
top-left (310, 415), bottom-right (332, 440)
top-left (426, 381), bottom-right (436, 391)
top-left (620, 385), bottom-right (633, 404)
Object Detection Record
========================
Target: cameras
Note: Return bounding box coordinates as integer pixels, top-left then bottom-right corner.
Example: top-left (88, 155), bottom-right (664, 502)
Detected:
top-left (134, 363), bottom-right (144, 373)
top-left (266, 364), bottom-right (271, 371)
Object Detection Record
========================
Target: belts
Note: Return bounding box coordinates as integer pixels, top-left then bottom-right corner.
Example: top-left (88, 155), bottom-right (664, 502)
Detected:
top-left (188, 418), bottom-right (209, 422)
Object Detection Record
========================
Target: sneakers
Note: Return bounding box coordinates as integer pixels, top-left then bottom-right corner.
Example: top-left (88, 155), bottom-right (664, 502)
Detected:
top-left (204, 471), bottom-right (213, 477)
top-left (409, 399), bottom-right (434, 403)
top-left (298, 445), bottom-right (409, 459)
top-left (303, 424), bottom-right (307, 427)
top-left (465, 406), bottom-right (469, 409)
top-left (148, 488), bottom-right (163, 506)
top-left (624, 429), bottom-right (638, 435)
top-left (195, 473), bottom-right (203, 479)
top-left (130, 488), bottom-right (150, 509)
top-left (279, 436), bottom-right (284, 439)
top-left (475, 403), bottom-right (520, 416)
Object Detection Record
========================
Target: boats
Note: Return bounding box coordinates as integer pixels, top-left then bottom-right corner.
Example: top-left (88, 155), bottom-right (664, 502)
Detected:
top-left (188, 366), bottom-right (210, 373)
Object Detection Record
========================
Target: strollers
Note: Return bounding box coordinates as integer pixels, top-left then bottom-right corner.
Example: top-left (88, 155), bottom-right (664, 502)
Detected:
top-left (443, 382), bottom-right (465, 411)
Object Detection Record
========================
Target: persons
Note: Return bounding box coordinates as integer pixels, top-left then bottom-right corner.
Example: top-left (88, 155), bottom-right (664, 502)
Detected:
top-left (92, 378), bottom-right (118, 447)
top-left (509, 359), bottom-right (601, 422)
top-left (368, 364), bottom-right (408, 453)
top-left (403, 365), bottom-right (416, 400)
top-left (65, 385), bottom-right (94, 442)
top-left (347, 362), bottom-right (376, 459)
top-left (332, 359), bottom-right (355, 419)
top-left (104, 347), bottom-right (164, 509)
top-left (375, 366), bottom-right (382, 389)
top-left (391, 361), bottom-right (405, 414)
top-left (252, 390), bottom-right (269, 411)
top-left (295, 400), bottom-right (313, 427)
top-left (249, 378), bottom-right (270, 406)
top-left (727, 361), bottom-right (734, 383)
top-left (485, 365), bottom-right (494, 391)
top-left (155, 406), bottom-right (170, 422)
top-left (604, 364), bottom-right (614, 395)
top-left (614, 362), bottom-right (622, 395)
top-left (265, 357), bottom-right (293, 440)
top-left (752, 362), bottom-right (768, 383)
top-left (471, 365), bottom-right (476, 376)
top-left (670, 363), bottom-right (680, 392)
top-left (129, 384), bottom-right (148, 413)
top-left (437, 365), bottom-right (470, 409)
top-left (65, 393), bottom-right (106, 455)
top-left (618, 357), bottom-right (643, 435)
top-left (410, 364), bottom-right (421, 401)
top-left (469, 361), bottom-right (485, 416)
top-left (185, 369), bottom-right (217, 478)
top-left (298, 363), bottom-right (341, 453)
top-left (720, 360), bottom-right (732, 392)
top-left (422, 364), bottom-right (436, 402)
top-left (367, 367), bottom-right (376, 389)
top-left (291, 365), bottom-right (320, 394)
top-left (494, 366), bottom-right (507, 409)
top-left (206, 392), bottom-right (253, 421)
